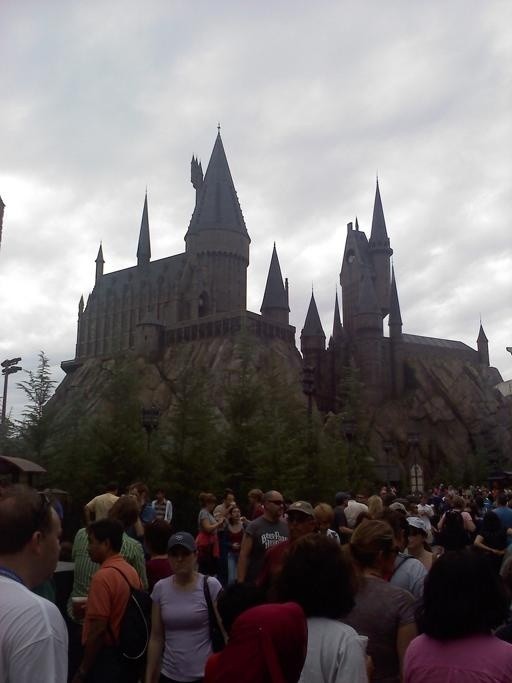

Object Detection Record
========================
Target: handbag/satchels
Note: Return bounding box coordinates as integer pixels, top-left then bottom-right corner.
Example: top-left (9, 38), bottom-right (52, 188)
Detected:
top-left (202, 575), bottom-right (227, 653)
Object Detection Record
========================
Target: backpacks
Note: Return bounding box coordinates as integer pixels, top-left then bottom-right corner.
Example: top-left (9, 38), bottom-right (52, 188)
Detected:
top-left (442, 508), bottom-right (465, 550)
top-left (98, 566), bottom-right (153, 661)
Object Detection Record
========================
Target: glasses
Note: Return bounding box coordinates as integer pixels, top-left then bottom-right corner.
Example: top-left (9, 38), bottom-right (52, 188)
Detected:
top-left (267, 499), bottom-right (285, 505)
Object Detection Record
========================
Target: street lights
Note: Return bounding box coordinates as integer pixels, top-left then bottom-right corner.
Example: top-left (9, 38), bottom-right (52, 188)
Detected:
top-left (301, 364), bottom-right (317, 447)
top-left (382, 439), bottom-right (395, 487)
top-left (1, 357), bottom-right (22, 424)
top-left (137, 405), bottom-right (161, 451)
top-left (406, 430), bottom-right (423, 492)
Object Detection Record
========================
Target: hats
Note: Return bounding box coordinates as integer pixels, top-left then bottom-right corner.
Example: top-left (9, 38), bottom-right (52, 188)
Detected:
top-left (167, 532), bottom-right (197, 554)
top-left (286, 501), bottom-right (315, 518)
top-left (335, 492), bottom-right (351, 504)
top-left (405, 517), bottom-right (428, 534)
top-left (389, 502), bottom-right (407, 514)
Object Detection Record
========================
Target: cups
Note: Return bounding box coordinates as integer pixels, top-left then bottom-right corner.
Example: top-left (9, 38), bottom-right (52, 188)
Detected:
top-left (359, 635), bottom-right (369, 658)
top-left (71, 596), bottom-right (89, 620)
